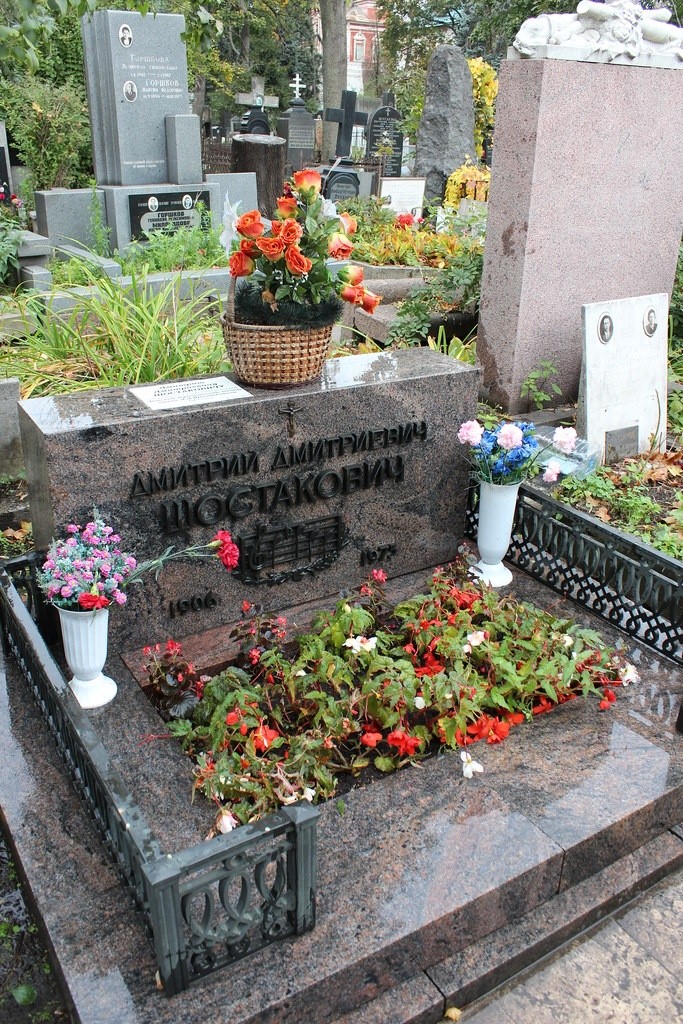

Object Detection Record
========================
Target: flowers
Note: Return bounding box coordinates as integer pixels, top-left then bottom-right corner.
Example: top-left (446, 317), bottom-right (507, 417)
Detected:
top-left (35, 501), bottom-right (240, 626)
top-left (219, 171), bottom-right (384, 328)
top-left (457, 418), bottom-right (578, 484)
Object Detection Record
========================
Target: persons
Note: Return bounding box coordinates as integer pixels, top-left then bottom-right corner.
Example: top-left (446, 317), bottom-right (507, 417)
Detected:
top-left (601, 316), bottom-right (612, 341)
top-left (148, 199), bottom-right (159, 210)
top-left (120, 27), bottom-right (133, 47)
top-left (182, 197), bottom-right (193, 209)
top-left (124, 82), bottom-right (138, 102)
top-left (645, 309), bottom-right (659, 335)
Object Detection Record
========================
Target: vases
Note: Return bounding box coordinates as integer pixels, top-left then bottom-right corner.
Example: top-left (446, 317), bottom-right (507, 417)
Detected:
top-left (49, 595), bottom-right (117, 709)
top-left (456, 475), bottom-right (578, 587)
